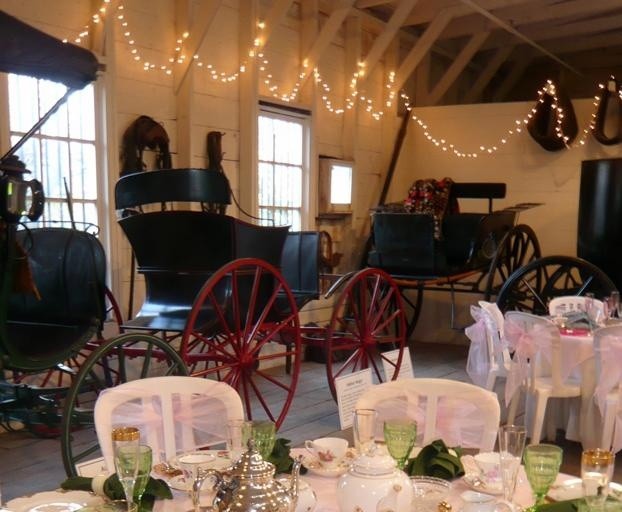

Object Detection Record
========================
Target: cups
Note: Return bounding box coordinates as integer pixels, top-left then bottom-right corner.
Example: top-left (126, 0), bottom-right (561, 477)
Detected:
top-left (554, 289), bottom-right (621, 332)
top-left (496, 423), bottom-right (527, 500)
top-left (382, 423), bottom-right (416, 467)
top-left (523, 443), bottom-right (564, 511)
top-left (305, 437), bottom-right (355, 462)
top-left (116, 444), bottom-right (149, 511)
top-left (580, 448), bottom-right (617, 509)
top-left (353, 407), bottom-right (379, 454)
top-left (223, 419), bottom-right (277, 463)
top-left (407, 474), bottom-right (452, 512)
top-left (111, 425), bottom-right (141, 506)
top-left (177, 453), bottom-right (212, 485)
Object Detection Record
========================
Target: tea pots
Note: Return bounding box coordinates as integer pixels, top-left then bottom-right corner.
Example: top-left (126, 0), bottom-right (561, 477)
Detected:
top-left (336, 434), bottom-right (416, 512)
top-left (278, 477), bottom-right (318, 511)
top-left (459, 489), bottom-right (515, 511)
top-left (189, 435), bottom-right (305, 512)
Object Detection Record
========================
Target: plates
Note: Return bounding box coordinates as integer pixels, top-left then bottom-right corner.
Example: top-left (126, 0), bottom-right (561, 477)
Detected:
top-left (300, 452), bottom-right (355, 478)
top-left (166, 472), bottom-right (211, 493)
top-left (167, 450), bottom-right (236, 469)
top-left (2, 491), bottom-right (109, 511)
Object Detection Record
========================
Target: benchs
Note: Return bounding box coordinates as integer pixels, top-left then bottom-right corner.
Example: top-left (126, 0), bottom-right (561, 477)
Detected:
top-left (114, 166), bottom-right (291, 332)
top-left (439, 182), bottom-right (519, 271)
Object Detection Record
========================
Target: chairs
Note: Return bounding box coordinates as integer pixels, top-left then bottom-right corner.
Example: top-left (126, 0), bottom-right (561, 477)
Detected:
top-left (90, 375), bottom-right (246, 476)
top-left (464, 294), bottom-right (621, 458)
top-left (353, 376), bottom-right (503, 451)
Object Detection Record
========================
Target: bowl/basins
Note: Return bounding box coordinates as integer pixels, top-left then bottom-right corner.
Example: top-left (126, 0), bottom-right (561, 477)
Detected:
top-left (472, 450), bottom-right (512, 488)
